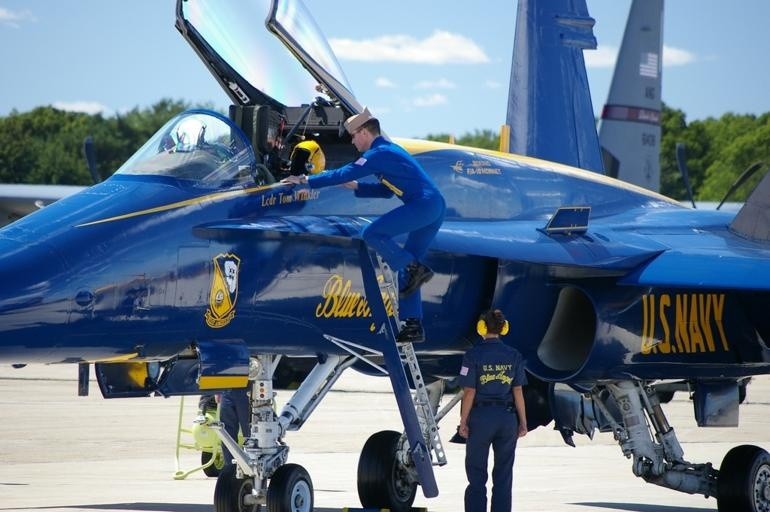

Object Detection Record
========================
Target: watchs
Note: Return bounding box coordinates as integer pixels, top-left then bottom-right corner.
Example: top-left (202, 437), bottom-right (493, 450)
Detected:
top-left (298, 173), bottom-right (305, 184)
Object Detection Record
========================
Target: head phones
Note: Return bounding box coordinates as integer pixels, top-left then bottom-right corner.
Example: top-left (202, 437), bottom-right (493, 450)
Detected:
top-left (477, 309), bottom-right (509, 336)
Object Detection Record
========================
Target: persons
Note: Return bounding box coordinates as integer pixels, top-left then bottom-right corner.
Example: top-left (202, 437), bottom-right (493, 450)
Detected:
top-left (459, 308), bottom-right (528, 512)
top-left (215, 393), bottom-right (252, 467)
top-left (277, 108), bottom-right (447, 345)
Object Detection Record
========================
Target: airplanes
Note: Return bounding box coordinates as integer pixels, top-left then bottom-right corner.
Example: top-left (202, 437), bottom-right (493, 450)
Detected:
top-left (0, 0), bottom-right (770, 512)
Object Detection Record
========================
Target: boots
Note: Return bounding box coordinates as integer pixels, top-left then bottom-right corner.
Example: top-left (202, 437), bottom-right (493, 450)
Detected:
top-left (398, 261), bottom-right (434, 298)
top-left (395, 318), bottom-right (426, 346)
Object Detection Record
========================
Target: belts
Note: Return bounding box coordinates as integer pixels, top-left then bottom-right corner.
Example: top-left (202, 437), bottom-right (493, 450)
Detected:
top-left (472, 400), bottom-right (515, 408)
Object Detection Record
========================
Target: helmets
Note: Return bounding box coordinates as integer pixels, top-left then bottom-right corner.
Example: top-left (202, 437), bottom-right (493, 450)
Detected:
top-left (290, 140), bottom-right (325, 173)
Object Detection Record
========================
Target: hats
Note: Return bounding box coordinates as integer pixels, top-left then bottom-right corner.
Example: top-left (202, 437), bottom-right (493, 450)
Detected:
top-left (343, 105), bottom-right (374, 136)
